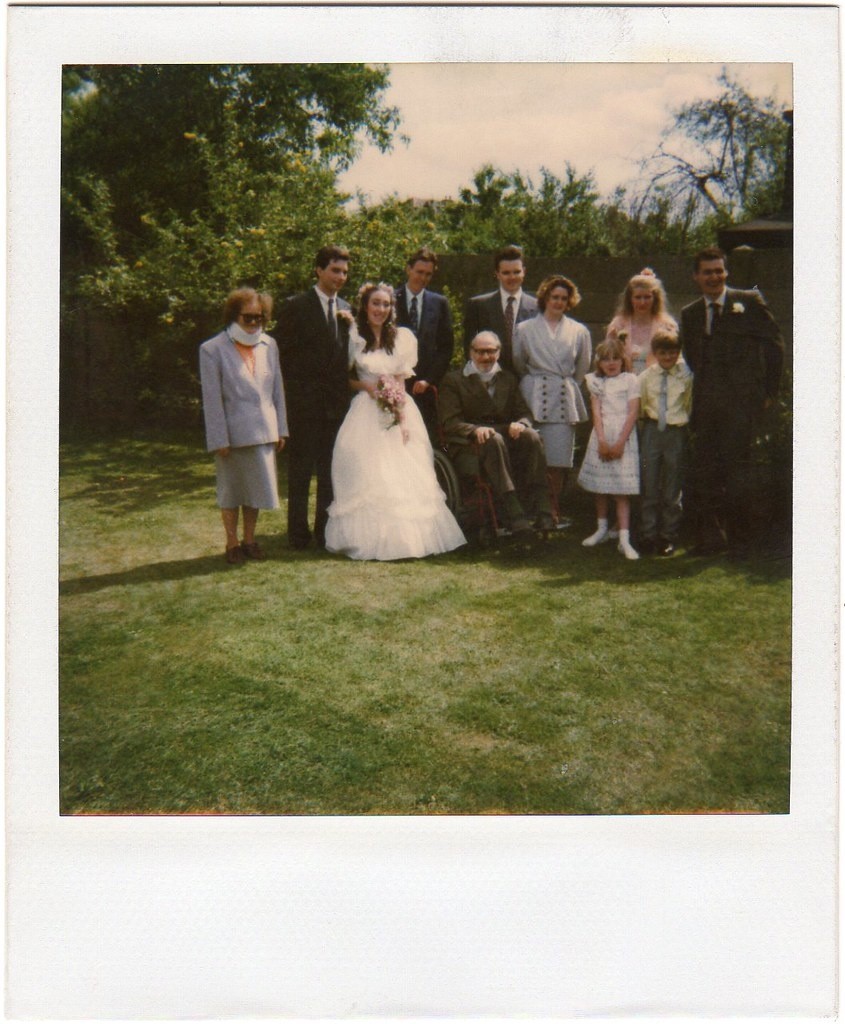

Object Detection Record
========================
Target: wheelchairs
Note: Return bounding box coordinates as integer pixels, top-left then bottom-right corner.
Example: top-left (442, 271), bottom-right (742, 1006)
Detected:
top-left (423, 383), bottom-right (560, 563)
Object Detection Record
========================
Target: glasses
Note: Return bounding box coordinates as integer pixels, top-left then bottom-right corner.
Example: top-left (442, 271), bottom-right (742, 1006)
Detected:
top-left (239, 312), bottom-right (264, 323)
top-left (470, 345), bottom-right (500, 355)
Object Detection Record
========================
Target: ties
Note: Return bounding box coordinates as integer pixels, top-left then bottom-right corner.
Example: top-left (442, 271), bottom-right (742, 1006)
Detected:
top-left (410, 297), bottom-right (418, 329)
top-left (505, 297), bottom-right (516, 345)
top-left (708, 303), bottom-right (721, 339)
top-left (658, 375), bottom-right (667, 432)
top-left (327, 299), bottom-right (344, 357)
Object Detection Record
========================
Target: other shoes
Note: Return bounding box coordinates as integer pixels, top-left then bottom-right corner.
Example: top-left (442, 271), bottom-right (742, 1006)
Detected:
top-left (287, 537), bottom-right (304, 551)
top-left (225, 542), bottom-right (246, 564)
top-left (617, 541), bottom-right (640, 561)
top-left (509, 517), bottom-right (531, 535)
top-left (657, 536), bottom-right (677, 557)
top-left (534, 515), bottom-right (556, 533)
top-left (243, 542), bottom-right (266, 560)
top-left (581, 531), bottom-right (610, 546)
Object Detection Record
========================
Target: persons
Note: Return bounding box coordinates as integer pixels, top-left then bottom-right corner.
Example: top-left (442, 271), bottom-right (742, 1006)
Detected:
top-left (682, 250), bottom-right (786, 557)
top-left (464, 244), bottom-right (541, 383)
top-left (198, 286), bottom-right (291, 563)
top-left (272, 245), bottom-right (356, 548)
top-left (606, 268), bottom-right (680, 540)
top-left (325, 282), bottom-right (469, 561)
top-left (577, 337), bottom-right (642, 561)
top-left (392, 248), bottom-right (456, 447)
top-left (439, 330), bottom-right (558, 534)
top-left (637, 331), bottom-right (693, 555)
top-left (512, 275), bottom-right (592, 526)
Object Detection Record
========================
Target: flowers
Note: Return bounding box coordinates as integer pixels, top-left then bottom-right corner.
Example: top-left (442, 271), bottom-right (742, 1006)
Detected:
top-left (732, 302), bottom-right (745, 315)
top-left (337, 311), bottom-right (353, 327)
top-left (375, 374), bottom-right (410, 430)
